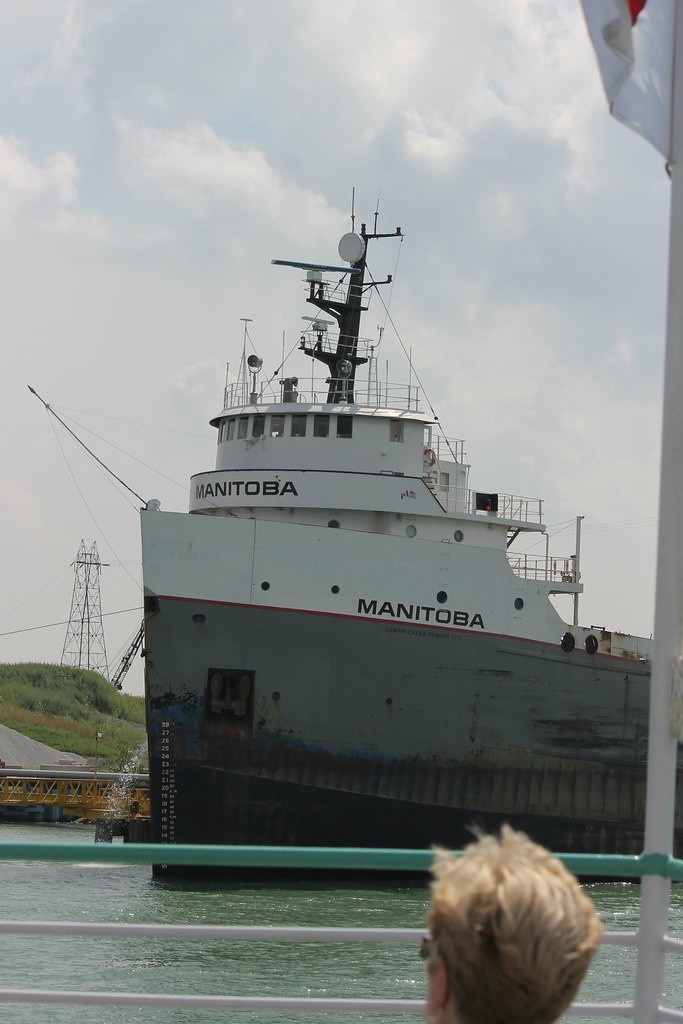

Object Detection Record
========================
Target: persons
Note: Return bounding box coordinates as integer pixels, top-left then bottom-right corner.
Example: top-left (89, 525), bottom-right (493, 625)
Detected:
top-left (424, 827), bottom-right (602, 1024)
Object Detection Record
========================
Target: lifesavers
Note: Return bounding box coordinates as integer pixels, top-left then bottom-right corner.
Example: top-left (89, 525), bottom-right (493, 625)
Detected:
top-left (424, 448), bottom-right (436, 466)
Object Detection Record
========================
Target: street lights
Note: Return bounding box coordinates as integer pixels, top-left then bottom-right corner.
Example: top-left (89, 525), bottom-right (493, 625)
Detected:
top-left (93, 731), bottom-right (104, 780)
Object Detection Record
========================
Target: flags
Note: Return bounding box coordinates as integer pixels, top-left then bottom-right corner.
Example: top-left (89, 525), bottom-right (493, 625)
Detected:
top-left (580, 0), bottom-right (675, 160)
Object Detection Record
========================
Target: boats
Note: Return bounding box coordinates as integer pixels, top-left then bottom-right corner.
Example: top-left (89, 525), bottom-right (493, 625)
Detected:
top-left (133, 178), bottom-right (683, 892)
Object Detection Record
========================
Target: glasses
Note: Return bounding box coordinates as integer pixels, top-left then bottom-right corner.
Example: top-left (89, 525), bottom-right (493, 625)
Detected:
top-left (420, 939), bottom-right (436, 958)
